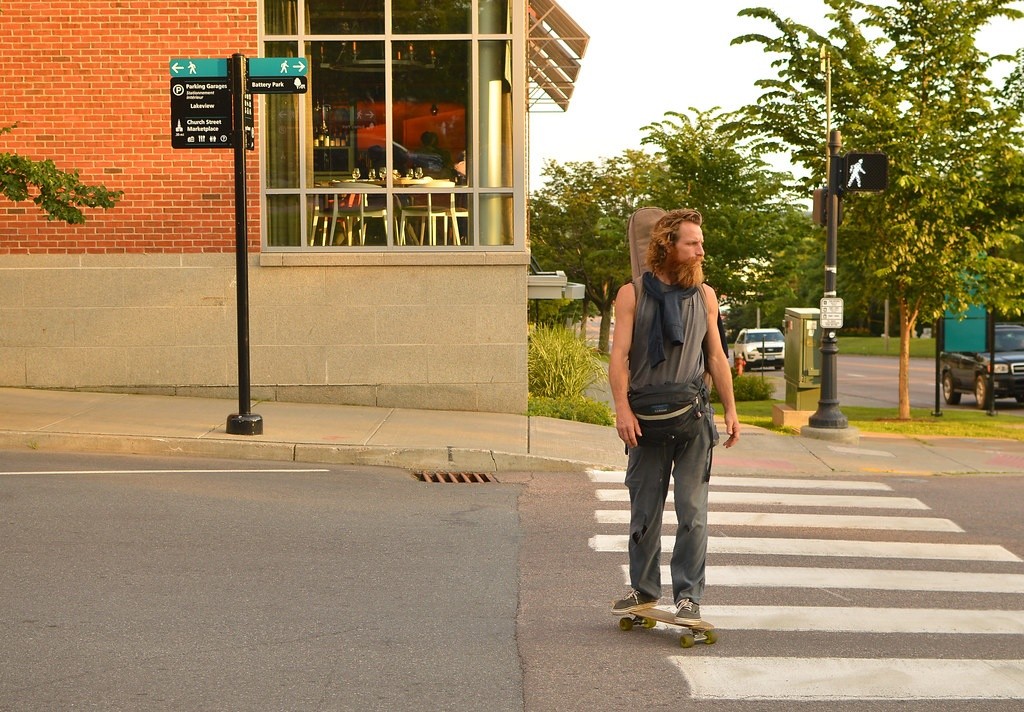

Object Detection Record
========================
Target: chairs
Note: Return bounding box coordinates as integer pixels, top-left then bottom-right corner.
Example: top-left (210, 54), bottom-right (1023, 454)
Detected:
top-left (357, 189), bottom-right (392, 244)
top-left (418, 184), bottom-right (469, 245)
top-left (311, 183), bottom-right (354, 245)
top-left (399, 181), bottom-right (461, 246)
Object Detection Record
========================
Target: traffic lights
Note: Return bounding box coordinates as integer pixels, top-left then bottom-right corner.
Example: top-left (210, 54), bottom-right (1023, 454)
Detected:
top-left (839, 153), bottom-right (888, 192)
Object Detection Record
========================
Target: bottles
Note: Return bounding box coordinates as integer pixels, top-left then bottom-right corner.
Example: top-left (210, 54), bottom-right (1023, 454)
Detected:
top-left (314, 122), bottom-right (349, 147)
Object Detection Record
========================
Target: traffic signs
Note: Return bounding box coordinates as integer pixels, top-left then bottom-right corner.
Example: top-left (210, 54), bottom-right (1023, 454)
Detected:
top-left (170, 57), bottom-right (232, 77)
top-left (246, 57), bottom-right (308, 76)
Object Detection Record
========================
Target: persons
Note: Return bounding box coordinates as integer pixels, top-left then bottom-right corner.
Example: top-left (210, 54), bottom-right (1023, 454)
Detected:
top-left (606, 209), bottom-right (741, 623)
top-left (413, 131), bottom-right (456, 178)
top-left (356, 145), bottom-right (385, 179)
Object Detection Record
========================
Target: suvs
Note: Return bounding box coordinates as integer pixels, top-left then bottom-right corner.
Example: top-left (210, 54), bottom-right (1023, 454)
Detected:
top-left (733, 328), bottom-right (785, 372)
top-left (939, 324), bottom-right (1024, 410)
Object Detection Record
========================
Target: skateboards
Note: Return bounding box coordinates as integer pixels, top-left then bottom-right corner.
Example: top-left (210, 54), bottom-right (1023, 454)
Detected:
top-left (617, 608), bottom-right (718, 648)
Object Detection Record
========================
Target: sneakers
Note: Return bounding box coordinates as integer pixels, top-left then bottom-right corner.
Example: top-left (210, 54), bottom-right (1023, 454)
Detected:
top-left (611, 589), bottom-right (658, 616)
top-left (674, 598), bottom-right (702, 626)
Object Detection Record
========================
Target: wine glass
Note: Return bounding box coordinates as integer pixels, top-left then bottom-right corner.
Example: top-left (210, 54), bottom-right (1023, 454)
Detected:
top-left (414, 166), bottom-right (423, 179)
top-left (352, 167), bottom-right (360, 181)
top-left (368, 168), bottom-right (376, 181)
top-left (379, 167), bottom-right (386, 180)
top-left (393, 169), bottom-right (401, 180)
top-left (406, 168), bottom-right (414, 180)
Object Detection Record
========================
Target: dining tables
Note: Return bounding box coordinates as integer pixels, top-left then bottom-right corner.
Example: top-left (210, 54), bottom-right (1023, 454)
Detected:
top-left (331, 179), bottom-right (462, 245)
